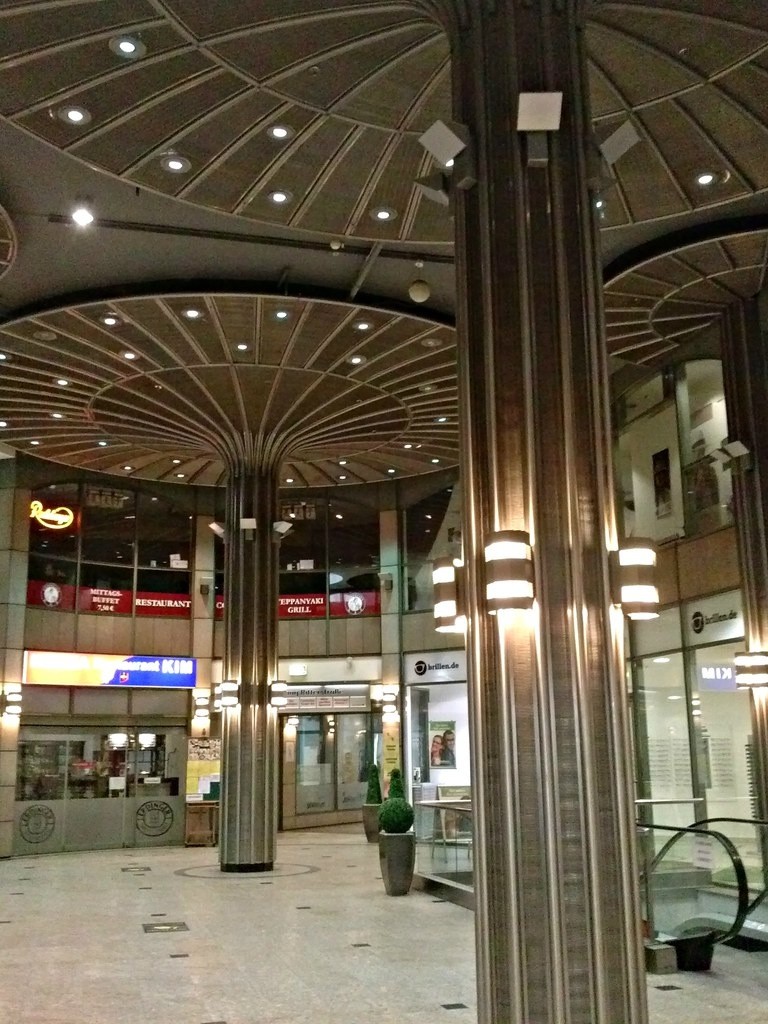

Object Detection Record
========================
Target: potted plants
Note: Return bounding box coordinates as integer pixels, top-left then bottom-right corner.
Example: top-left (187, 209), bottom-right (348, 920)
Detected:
top-left (362, 765), bottom-right (383, 843)
top-left (377, 797), bottom-right (415, 896)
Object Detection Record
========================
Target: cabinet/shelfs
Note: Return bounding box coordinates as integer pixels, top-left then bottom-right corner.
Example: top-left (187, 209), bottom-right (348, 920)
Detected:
top-left (185, 801), bottom-right (219, 848)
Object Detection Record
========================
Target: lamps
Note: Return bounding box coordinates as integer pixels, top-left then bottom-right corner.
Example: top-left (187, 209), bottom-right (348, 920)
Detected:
top-left (240, 518), bottom-right (257, 541)
top-left (482, 530), bottom-right (535, 617)
top-left (711, 439), bottom-right (753, 475)
top-left (209, 522), bottom-right (227, 535)
top-left (221, 680), bottom-right (239, 706)
top-left (418, 119), bottom-right (477, 189)
top-left (595, 120), bottom-right (639, 165)
top-left (408, 259), bottom-right (431, 303)
top-left (414, 172), bottom-right (450, 206)
top-left (383, 692), bottom-right (396, 713)
top-left (433, 557), bottom-right (465, 634)
top-left (269, 681), bottom-right (287, 706)
top-left (272, 520), bottom-right (293, 534)
top-left (328, 721), bottom-right (335, 734)
top-left (288, 715), bottom-right (298, 725)
top-left (0, 690), bottom-right (22, 718)
top-left (214, 685), bottom-right (222, 708)
top-left (735, 649), bottom-right (767, 690)
top-left (195, 697), bottom-right (209, 716)
top-left (200, 577), bottom-right (213, 594)
top-left (281, 529), bottom-right (295, 539)
top-left (378, 571), bottom-right (393, 590)
top-left (516, 90), bottom-right (563, 166)
top-left (609, 537), bottom-right (660, 621)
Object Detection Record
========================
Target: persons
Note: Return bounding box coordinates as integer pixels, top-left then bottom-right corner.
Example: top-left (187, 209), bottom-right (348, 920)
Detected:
top-left (430, 730), bottom-right (455, 765)
top-left (408, 576), bottom-right (418, 611)
top-left (690, 438), bottom-right (722, 531)
